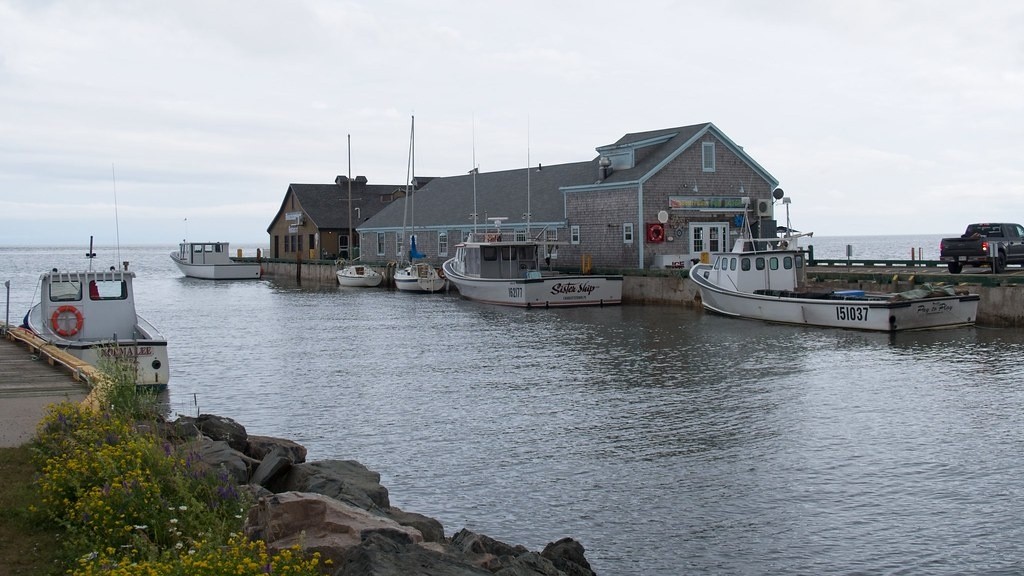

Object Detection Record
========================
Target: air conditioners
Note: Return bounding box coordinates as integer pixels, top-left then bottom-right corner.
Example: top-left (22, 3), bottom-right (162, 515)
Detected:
top-left (295, 215), bottom-right (303, 225)
top-left (753, 199), bottom-right (773, 216)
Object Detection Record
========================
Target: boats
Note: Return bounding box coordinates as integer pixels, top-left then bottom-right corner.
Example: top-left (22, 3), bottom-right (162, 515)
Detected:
top-left (170, 239), bottom-right (261, 279)
top-left (394, 117), bottom-right (447, 292)
top-left (20, 160), bottom-right (169, 389)
top-left (689, 202), bottom-right (981, 333)
top-left (442, 123), bottom-right (624, 309)
top-left (335, 134), bottom-right (383, 287)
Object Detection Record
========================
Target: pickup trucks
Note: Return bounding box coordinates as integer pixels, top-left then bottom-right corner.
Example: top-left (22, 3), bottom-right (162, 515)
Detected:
top-left (938, 223), bottom-right (1024, 274)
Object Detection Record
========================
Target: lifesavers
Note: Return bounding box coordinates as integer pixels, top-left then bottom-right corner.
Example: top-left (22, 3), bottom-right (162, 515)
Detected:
top-left (438, 268), bottom-right (445, 279)
top-left (51, 305), bottom-right (83, 336)
top-left (648, 224), bottom-right (664, 242)
